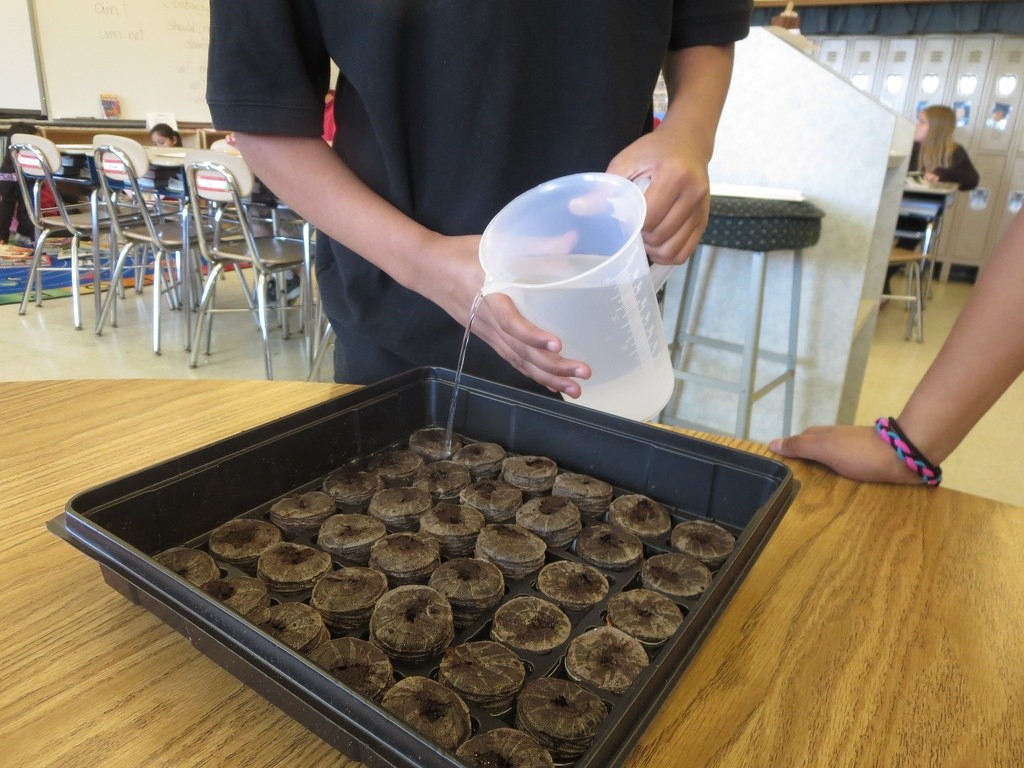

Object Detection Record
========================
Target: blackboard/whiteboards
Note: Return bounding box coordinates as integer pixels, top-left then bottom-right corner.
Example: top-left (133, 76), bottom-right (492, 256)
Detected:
top-left (26, 0), bottom-right (340, 130)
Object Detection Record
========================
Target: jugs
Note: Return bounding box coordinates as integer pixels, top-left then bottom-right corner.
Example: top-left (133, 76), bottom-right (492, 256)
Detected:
top-left (478, 170), bottom-right (678, 424)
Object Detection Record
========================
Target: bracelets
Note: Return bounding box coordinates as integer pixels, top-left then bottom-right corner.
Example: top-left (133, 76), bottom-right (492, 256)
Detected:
top-left (876, 416), bottom-right (944, 487)
top-left (887, 416), bottom-right (942, 477)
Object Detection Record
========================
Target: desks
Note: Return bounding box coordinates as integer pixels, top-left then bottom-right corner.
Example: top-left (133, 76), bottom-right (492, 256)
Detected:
top-left (899, 180), bottom-right (961, 341)
top-left (0, 380), bottom-right (1024, 768)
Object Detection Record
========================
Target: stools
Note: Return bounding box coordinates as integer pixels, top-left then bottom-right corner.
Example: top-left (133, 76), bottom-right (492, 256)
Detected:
top-left (657, 193), bottom-right (827, 443)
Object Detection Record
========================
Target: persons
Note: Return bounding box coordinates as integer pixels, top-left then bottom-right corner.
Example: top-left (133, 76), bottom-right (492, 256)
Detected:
top-left (150, 123), bottom-right (183, 201)
top-left (770, 205), bottom-right (1024, 485)
top-left (207, 0), bottom-right (754, 400)
top-left (881, 106), bottom-right (980, 307)
top-left (322, 90), bottom-right (337, 145)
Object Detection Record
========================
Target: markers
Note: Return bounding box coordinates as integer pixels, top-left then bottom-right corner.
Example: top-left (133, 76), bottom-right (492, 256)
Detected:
top-left (76, 116), bottom-right (96, 120)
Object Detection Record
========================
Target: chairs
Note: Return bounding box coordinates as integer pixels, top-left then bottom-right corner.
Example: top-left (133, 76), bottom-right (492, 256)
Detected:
top-left (184, 151), bottom-right (322, 380)
top-left (9, 133), bottom-right (146, 331)
top-left (94, 133), bottom-right (223, 359)
top-left (889, 249), bottom-right (935, 342)
